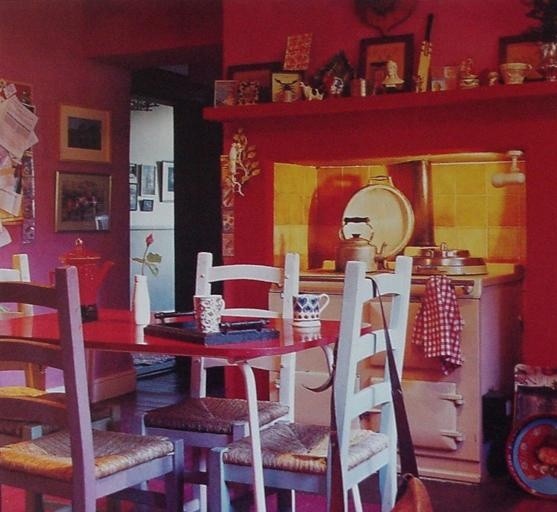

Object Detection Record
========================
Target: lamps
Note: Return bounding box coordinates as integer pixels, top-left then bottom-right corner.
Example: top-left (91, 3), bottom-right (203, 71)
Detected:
top-left (491, 150), bottom-right (525, 188)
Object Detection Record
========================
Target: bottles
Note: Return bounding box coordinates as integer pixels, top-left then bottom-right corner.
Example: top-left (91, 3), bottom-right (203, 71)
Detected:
top-left (535, 41), bottom-right (557, 82)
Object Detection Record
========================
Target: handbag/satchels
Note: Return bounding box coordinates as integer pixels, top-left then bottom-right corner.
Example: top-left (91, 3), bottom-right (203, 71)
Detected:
top-left (390, 472), bottom-right (432, 511)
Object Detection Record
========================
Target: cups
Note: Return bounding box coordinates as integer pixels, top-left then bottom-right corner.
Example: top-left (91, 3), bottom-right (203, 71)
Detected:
top-left (292, 293), bottom-right (330, 328)
top-left (499, 62), bottom-right (533, 86)
top-left (193, 295), bottom-right (226, 334)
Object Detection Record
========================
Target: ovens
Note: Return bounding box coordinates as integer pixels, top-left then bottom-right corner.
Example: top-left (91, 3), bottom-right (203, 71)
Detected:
top-left (268, 292), bottom-right (481, 487)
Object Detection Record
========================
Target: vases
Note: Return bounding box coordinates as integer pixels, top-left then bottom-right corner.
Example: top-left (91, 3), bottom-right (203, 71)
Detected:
top-left (132, 274), bottom-right (151, 325)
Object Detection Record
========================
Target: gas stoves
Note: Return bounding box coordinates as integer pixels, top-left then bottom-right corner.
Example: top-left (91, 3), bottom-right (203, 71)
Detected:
top-left (268, 260), bottom-right (515, 299)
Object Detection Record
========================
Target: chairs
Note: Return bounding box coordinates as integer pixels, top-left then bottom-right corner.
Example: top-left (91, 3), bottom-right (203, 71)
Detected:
top-left (207, 255), bottom-right (413, 512)
top-left (133, 252), bottom-right (301, 512)
top-left (0, 264), bottom-right (173, 512)
top-left (0, 254), bottom-right (122, 512)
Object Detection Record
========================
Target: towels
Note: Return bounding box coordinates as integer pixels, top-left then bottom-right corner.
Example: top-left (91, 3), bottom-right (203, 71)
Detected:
top-left (412, 276), bottom-right (463, 376)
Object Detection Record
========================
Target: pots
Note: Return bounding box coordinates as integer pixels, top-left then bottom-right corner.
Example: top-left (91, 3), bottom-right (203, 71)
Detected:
top-left (341, 175), bottom-right (416, 261)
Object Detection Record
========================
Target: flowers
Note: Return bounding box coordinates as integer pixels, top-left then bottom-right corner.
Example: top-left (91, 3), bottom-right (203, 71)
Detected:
top-left (131, 232), bottom-right (162, 277)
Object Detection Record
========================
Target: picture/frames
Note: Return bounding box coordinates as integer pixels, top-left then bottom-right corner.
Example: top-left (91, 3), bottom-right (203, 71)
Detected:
top-left (359, 34), bottom-right (414, 94)
top-left (161, 160), bottom-right (175, 204)
top-left (56, 171), bottom-right (114, 232)
top-left (228, 62), bottom-right (280, 89)
top-left (139, 165), bottom-right (156, 197)
top-left (142, 200), bottom-right (153, 209)
top-left (129, 183), bottom-right (136, 211)
top-left (129, 161), bottom-right (137, 184)
top-left (58, 103), bottom-right (112, 164)
top-left (499, 34), bottom-right (544, 83)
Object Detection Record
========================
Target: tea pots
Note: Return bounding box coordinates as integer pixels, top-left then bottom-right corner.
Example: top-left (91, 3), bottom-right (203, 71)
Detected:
top-left (334, 216), bottom-right (388, 273)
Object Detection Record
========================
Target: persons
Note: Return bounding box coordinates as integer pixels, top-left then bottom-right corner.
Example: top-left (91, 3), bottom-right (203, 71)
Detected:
top-left (381, 60), bottom-right (405, 85)
